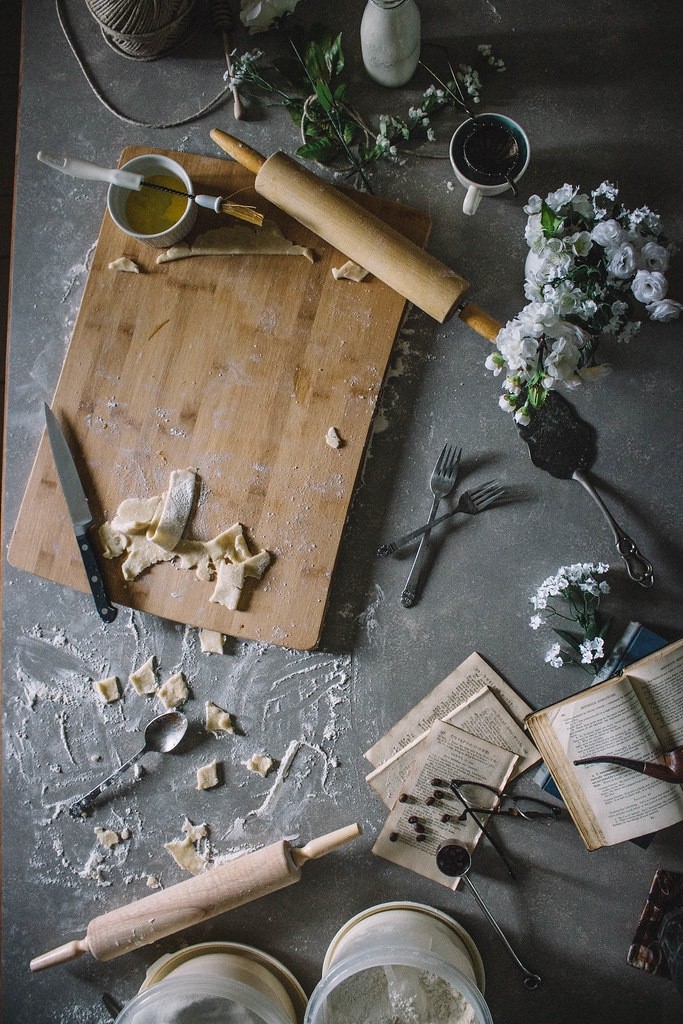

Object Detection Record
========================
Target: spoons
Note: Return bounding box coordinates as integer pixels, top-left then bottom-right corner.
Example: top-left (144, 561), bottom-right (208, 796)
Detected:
top-left (67, 710), bottom-right (190, 818)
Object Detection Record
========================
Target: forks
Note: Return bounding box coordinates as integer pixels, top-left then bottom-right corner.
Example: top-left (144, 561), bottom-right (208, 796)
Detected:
top-left (401, 443), bottom-right (462, 608)
top-left (376, 478), bottom-right (506, 558)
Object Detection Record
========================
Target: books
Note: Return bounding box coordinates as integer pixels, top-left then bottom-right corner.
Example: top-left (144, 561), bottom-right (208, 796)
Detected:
top-left (525, 623), bottom-right (683, 853)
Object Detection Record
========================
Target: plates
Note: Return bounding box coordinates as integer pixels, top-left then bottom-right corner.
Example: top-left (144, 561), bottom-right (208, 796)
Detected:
top-left (322, 900), bottom-right (486, 995)
top-left (138, 942), bottom-right (308, 1024)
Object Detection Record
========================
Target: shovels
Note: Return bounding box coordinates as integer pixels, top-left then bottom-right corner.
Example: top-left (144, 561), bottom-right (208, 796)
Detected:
top-left (513, 390), bottom-right (655, 590)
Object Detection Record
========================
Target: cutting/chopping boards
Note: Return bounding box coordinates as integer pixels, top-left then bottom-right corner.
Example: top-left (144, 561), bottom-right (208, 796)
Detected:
top-left (4, 149), bottom-right (435, 650)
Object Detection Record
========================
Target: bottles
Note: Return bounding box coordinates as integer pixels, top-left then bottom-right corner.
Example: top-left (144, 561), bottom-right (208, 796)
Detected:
top-left (359, 0), bottom-right (421, 87)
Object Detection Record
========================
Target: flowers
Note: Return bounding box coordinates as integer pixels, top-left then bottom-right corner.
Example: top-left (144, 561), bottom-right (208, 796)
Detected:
top-left (338, 44), bottom-right (508, 182)
top-left (527, 561), bottom-right (614, 677)
top-left (222, 0), bottom-right (376, 197)
top-left (483, 180), bottom-right (683, 425)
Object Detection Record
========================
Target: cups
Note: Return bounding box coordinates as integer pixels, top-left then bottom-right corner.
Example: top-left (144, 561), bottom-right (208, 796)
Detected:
top-left (449, 113), bottom-right (530, 216)
top-left (303, 908), bottom-right (493, 1024)
top-left (106, 154), bottom-right (197, 247)
top-left (113, 956), bottom-right (295, 1023)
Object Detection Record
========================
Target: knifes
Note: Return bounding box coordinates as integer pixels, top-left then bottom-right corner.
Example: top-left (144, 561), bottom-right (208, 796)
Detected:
top-left (43, 400), bottom-right (117, 623)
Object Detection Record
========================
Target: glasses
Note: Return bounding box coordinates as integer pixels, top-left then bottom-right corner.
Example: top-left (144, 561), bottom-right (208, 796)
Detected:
top-left (449, 779), bottom-right (561, 882)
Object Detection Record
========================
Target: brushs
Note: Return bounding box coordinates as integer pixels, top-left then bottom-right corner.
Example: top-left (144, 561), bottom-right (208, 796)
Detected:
top-left (35, 151), bottom-right (266, 228)
